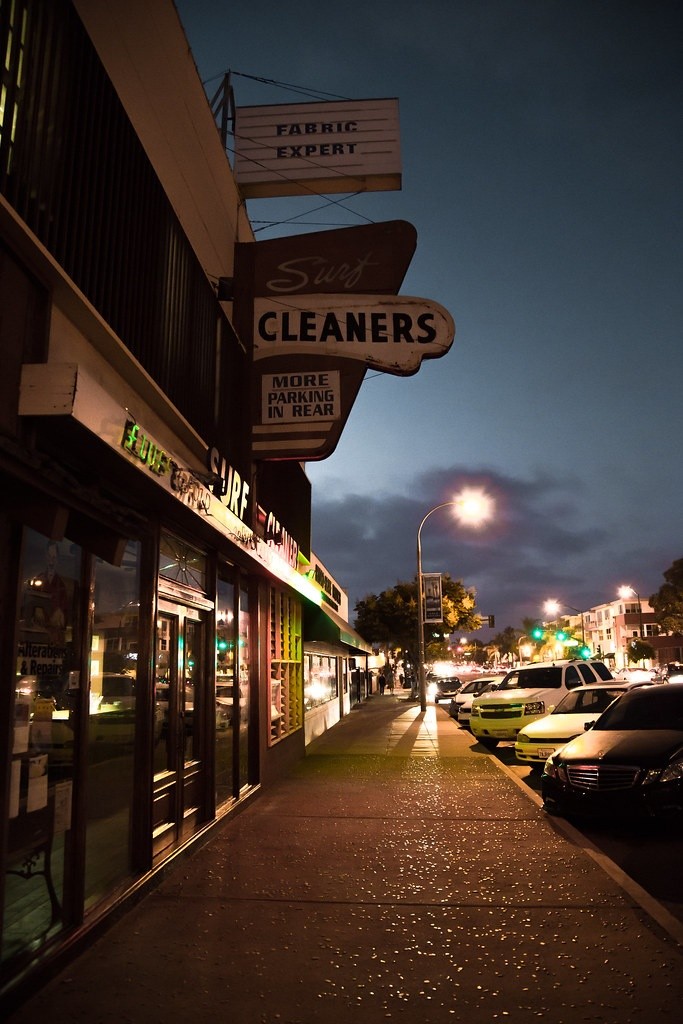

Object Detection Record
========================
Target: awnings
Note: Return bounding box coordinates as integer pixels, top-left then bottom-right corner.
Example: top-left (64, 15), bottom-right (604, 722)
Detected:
top-left (303, 600), bottom-right (373, 656)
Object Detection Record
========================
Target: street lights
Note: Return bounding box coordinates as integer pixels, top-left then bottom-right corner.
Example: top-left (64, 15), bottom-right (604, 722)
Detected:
top-left (619, 584), bottom-right (645, 667)
top-left (544, 599), bottom-right (587, 662)
top-left (417, 487), bottom-right (495, 712)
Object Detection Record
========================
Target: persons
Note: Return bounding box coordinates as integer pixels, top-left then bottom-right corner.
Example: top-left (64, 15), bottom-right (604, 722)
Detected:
top-left (399, 674), bottom-right (405, 685)
top-left (379, 673), bottom-right (386, 696)
top-left (387, 675), bottom-right (395, 696)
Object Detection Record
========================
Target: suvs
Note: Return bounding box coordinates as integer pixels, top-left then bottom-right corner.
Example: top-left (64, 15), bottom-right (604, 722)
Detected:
top-left (469, 659), bottom-right (614, 749)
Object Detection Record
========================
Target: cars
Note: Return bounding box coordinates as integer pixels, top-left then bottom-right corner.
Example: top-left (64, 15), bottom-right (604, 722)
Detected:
top-left (515, 680), bottom-right (656, 770)
top-left (614, 660), bottom-right (683, 684)
top-left (434, 676), bottom-right (465, 704)
top-left (451, 676), bottom-right (513, 727)
top-left (540, 681), bottom-right (682, 828)
top-left (38, 666), bottom-right (249, 758)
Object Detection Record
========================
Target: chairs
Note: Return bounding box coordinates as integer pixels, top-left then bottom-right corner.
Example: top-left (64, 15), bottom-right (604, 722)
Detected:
top-left (6, 749), bottom-right (62, 922)
